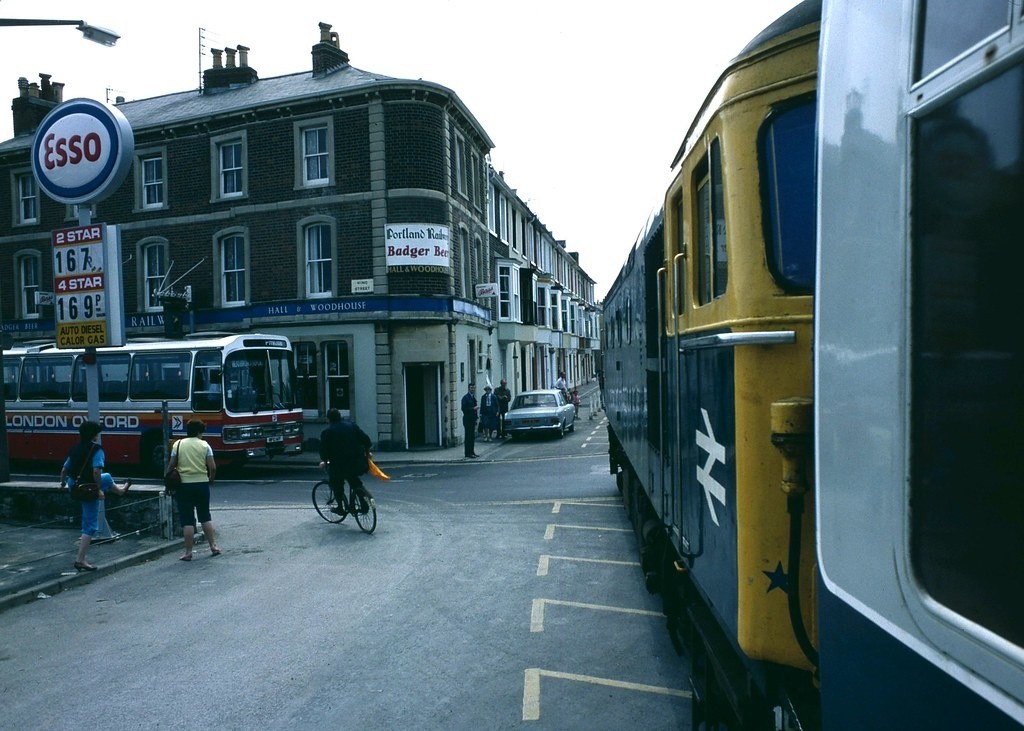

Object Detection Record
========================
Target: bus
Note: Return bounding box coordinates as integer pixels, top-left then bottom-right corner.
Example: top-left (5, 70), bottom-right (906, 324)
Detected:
top-left (0, 331), bottom-right (309, 479)
top-left (595, 1), bottom-right (1024, 728)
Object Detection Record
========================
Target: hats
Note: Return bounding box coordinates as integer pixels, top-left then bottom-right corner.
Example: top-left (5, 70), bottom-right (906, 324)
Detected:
top-left (79, 422), bottom-right (104, 437)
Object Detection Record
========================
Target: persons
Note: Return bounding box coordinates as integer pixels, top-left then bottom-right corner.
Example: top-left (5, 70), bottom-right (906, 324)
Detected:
top-left (479, 385), bottom-right (499, 442)
top-left (461, 383), bottom-right (480, 458)
top-left (552, 372), bottom-right (581, 419)
top-left (60, 421), bottom-right (132, 572)
top-left (319, 409), bottom-right (372, 515)
top-left (493, 379), bottom-right (511, 439)
top-left (164, 420), bottom-right (219, 561)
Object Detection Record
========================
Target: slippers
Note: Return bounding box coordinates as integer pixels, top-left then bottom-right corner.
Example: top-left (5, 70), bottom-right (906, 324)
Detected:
top-left (179, 553), bottom-right (192, 561)
top-left (211, 548), bottom-right (221, 555)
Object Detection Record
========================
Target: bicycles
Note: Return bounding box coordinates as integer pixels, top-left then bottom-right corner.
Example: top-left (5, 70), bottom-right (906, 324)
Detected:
top-left (311, 464), bottom-right (378, 535)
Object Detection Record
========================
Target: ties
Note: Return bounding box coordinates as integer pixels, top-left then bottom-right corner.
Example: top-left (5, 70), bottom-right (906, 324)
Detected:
top-left (487, 394), bottom-right (489, 404)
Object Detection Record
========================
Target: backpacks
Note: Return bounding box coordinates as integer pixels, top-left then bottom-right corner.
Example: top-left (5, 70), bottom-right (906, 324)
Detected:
top-left (343, 421), bottom-right (372, 477)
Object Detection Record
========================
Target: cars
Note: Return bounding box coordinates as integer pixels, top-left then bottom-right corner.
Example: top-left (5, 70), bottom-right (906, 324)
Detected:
top-left (505, 390), bottom-right (576, 441)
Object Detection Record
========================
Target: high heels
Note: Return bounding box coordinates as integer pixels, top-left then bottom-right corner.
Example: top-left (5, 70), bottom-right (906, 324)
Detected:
top-left (123, 478), bottom-right (131, 495)
top-left (74, 561), bottom-right (97, 572)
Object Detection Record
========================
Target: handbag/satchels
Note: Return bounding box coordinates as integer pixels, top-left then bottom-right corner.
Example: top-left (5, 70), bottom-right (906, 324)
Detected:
top-left (70, 482), bottom-right (98, 501)
top-left (478, 419), bottom-right (484, 433)
top-left (164, 441), bottom-right (180, 489)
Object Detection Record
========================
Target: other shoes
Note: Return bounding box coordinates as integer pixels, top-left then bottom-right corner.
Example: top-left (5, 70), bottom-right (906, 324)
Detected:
top-left (483, 436), bottom-right (488, 441)
top-left (465, 453), bottom-right (479, 458)
top-left (488, 437), bottom-right (492, 442)
